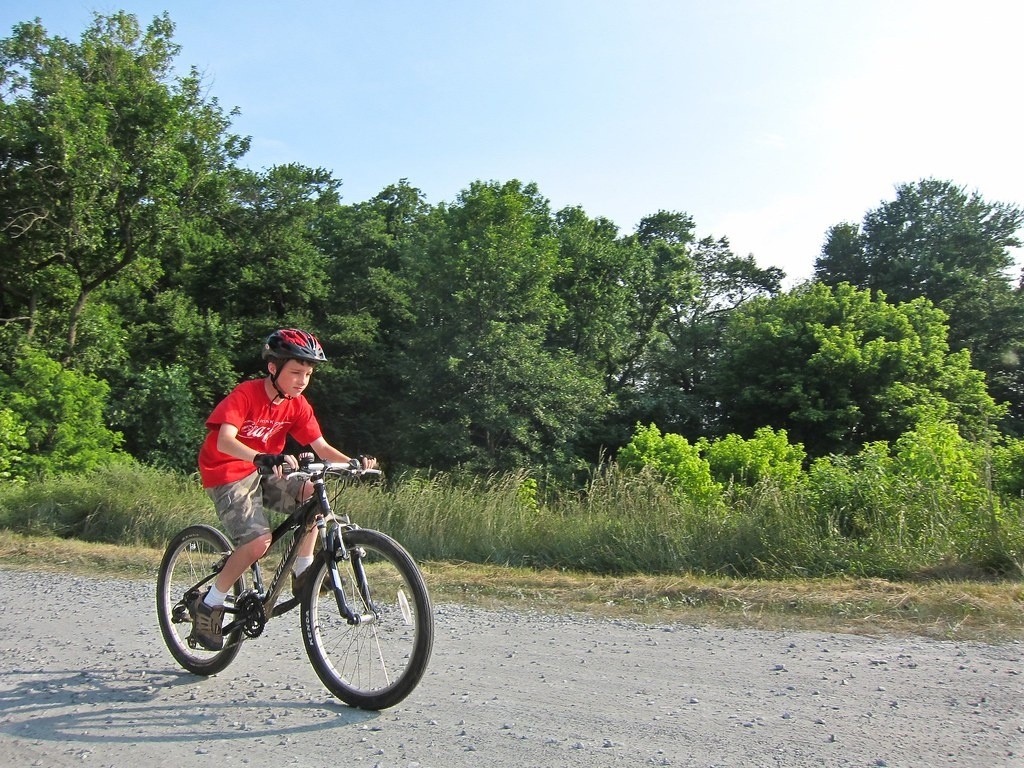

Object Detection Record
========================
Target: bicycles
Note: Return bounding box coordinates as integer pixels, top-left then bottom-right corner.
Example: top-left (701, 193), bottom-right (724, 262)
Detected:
top-left (156, 452), bottom-right (435, 711)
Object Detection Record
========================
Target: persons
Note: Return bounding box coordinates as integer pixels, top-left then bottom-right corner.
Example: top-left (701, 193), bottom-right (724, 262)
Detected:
top-left (187, 329), bottom-right (376, 651)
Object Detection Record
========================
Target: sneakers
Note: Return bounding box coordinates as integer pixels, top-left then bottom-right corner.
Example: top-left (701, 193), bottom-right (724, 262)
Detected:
top-left (189, 586), bottom-right (225, 651)
top-left (291, 565), bottom-right (346, 597)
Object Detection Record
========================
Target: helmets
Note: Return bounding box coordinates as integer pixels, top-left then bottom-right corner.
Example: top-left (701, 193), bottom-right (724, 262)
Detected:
top-left (262, 329), bottom-right (328, 362)
